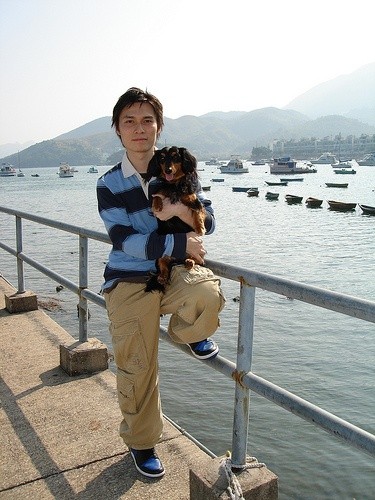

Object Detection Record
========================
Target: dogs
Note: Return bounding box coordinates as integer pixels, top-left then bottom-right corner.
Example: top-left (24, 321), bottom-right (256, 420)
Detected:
top-left (143, 144), bottom-right (208, 295)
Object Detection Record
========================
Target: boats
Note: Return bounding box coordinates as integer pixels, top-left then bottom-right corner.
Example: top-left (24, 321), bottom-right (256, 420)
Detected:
top-left (358, 203), bottom-right (375, 217)
top-left (212, 178), bottom-right (224, 182)
top-left (30, 161), bottom-right (99, 178)
top-left (202, 185), bottom-right (211, 191)
top-left (325, 199), bottom-right (357, 212)
top-left (219, 159), bottom-right (249, 173)
top-left (280, 177), bottom-right (304, 182)
top-left (247, 188), bottom-right (260, 197)
top-left (266, 191), bottom-right (279, 201)
top-left (333, 169), bottom-right (356, 174)
top-left (250, 150), bottom-right (353, 174)
top-left (205, 158), bottom-right (219, 166)
top-left (0, 162), bottom-right (16, 176)
top-left (285, 194), bottom-right (303, 205)
top-left (325, 183), bottom-right (348, 188)
top-left (355, 153), bottom-right (375, 166)
top-left (232, 187), bottom-right (257, 192)
top-left (265, 181), bottom-right (288, 186)
top-left (306, 197), bottom-right (324, 208)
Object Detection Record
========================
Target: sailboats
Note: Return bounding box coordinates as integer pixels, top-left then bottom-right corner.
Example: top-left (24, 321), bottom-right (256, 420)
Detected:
top-left (17, 149), bottom-right (25, 177)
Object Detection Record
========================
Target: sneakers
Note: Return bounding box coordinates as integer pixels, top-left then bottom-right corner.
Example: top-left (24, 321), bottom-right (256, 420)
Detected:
top-left (186, 337), bottom-right (219, 359)
top-left (129, 447), bottom-right (165, 477)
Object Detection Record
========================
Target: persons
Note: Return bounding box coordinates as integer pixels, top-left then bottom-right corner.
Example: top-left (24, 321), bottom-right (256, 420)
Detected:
top-left (95, 86), bottom-right (227, 477)
